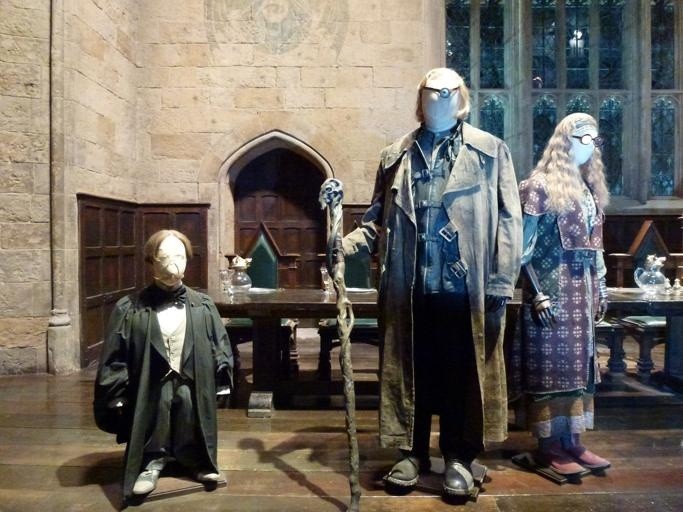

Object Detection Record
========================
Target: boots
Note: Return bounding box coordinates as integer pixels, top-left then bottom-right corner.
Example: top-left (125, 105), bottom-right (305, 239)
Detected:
top-left (534, 435), bottom-right (586, 478)
top-left (560, 432), bottom-right (611, 470)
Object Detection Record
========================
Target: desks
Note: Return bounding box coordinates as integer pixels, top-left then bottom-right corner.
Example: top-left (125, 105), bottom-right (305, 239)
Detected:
top-left (193, 285), bottom-right (683, 430)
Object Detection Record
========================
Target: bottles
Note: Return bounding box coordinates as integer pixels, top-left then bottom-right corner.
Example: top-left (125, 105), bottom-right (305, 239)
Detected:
top-left (217, 263), bottom-right (253, 297)
top-left (662, 277), bottom-right (682, 296)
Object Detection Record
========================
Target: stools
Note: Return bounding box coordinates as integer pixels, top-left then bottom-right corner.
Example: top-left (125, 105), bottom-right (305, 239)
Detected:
top-left (315, 319), bottom-right (379, 380)
top-left (224, 317), bottom-right (300, 378)
top-left (594, 315), bottom-right (667, 384)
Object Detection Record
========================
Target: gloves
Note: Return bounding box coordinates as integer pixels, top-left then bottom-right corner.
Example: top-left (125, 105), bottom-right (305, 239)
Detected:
top-left (532, 296), bottom-right (557, 330)
top-left (592, 299), bottom-right (609, 325)
top-left (485, 293), bottom-right (508, 314)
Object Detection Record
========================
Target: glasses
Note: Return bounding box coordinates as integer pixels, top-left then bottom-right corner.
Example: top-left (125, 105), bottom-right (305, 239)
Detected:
top-left (571, 133), bottom-right (604, 148)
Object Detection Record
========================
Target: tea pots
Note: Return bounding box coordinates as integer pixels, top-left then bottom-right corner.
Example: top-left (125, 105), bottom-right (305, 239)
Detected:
top-left (633, 255), bottom-right (668, 294)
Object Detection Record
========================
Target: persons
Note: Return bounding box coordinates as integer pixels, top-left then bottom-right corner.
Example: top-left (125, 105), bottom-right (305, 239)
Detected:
top-left (339, 68), bottom-right (529, 505)
top-left (508, 112), bottom-right (615, 481)
top-left (90, 227), bottom-right (239, 497)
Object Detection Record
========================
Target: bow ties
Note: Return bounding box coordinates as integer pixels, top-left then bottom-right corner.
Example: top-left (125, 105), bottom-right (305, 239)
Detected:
top-left (150, 293), bottom-right (186, 306)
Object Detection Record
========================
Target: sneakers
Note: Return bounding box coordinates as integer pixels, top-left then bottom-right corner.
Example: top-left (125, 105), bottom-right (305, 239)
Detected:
top-left (191, 465), bottom-right (227, 485)
top-left (131, 452), bottom-right (169, 495)
top-left (380, 449), bottom-right (430, 489)
top-left (441, 451), bottom-right (476, 498)
